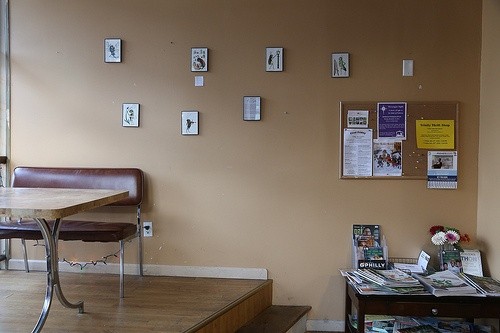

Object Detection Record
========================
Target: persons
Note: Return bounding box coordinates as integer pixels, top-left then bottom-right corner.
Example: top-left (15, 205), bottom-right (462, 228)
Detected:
top-left (436, 157), bottom-right (442, 167)
top-left (362, 227), bottom-right (379, 247)
top-left (377, 150), bottom-right (398, 167)
top-left (443, 262), bottom-right (448, 271)
top-left (450, 258), bottom-right (460, 270)
top-left (455, 260), bottom-right (462, 267)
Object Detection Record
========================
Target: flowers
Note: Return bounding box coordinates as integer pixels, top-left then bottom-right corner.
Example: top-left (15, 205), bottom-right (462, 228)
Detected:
top-left (430, 225), bottom-right (471, 246)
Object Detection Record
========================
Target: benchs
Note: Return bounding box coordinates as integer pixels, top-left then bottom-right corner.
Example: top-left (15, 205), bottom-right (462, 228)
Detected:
top-left (0, 167), bottom-right (144, 298)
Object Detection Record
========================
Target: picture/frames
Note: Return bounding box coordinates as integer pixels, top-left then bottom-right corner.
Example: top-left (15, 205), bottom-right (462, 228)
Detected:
top-left (104, 38), bottom-right (121, 63)
top-left (122, 103), bottom-right (139, 127)
top-left (265, 47), bottom-right (283, 72)
top-left (191, 48), bottom-right (208, 71)
top-left (243, 96), bottom-right (261, 121)
top-left (331, 53), bottom-right (350, 78)
top-left (182, 111), bottom-right (199, 136)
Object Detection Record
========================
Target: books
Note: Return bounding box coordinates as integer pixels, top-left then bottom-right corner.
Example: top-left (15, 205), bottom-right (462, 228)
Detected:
top-left (338, 224), bottom-right (500, 297)
top-left (348, 307), bottom-right (498, 333)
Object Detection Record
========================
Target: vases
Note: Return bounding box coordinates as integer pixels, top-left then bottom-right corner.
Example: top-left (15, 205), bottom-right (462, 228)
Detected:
top-left (438, 245), bottom-right (454, 269)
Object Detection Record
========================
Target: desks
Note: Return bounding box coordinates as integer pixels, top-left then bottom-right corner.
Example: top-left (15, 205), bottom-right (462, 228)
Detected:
top-left (0, 187), bottom-right (129, 333)
top-left (344, 277), bottom-right (500, 333)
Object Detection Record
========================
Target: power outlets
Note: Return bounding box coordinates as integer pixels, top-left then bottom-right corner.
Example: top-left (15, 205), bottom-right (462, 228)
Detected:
top-left (143, 222), bottom-right (152, 237)
